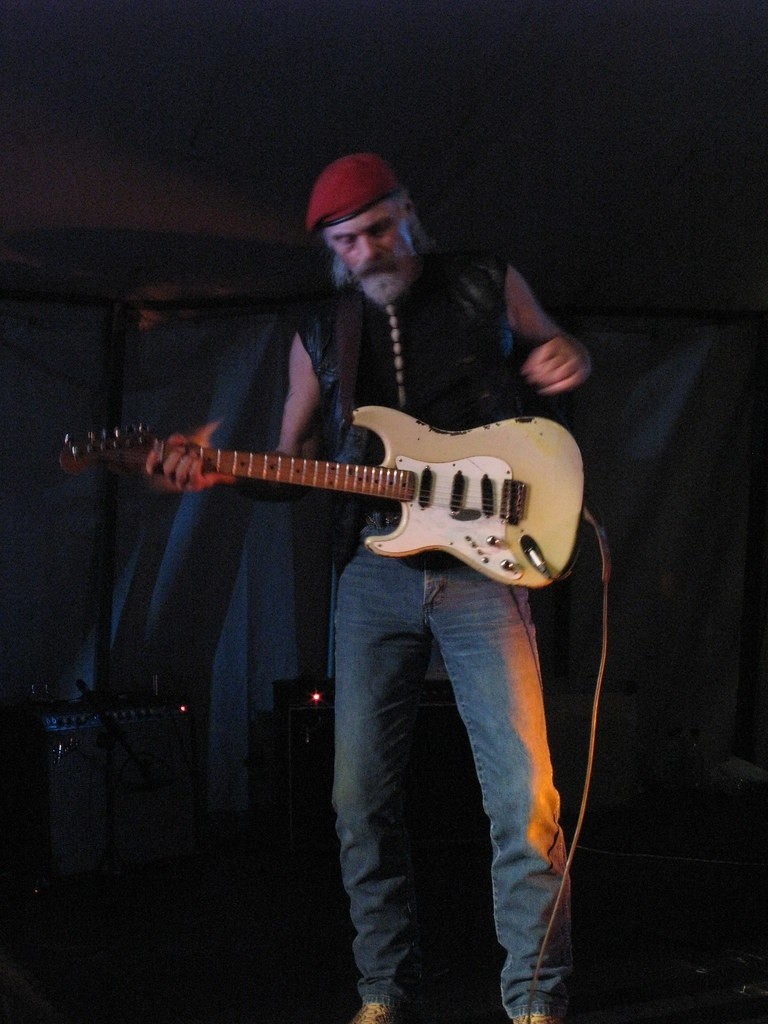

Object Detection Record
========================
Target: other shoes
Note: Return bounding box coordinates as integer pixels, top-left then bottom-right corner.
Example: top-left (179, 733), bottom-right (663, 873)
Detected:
top-left (511, 1009), bottom-right (561, 1024)
top-left (350, 1001), bottom-right (396, 1024)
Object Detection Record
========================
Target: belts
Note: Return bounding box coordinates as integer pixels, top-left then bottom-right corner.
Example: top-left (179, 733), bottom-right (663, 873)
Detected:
top-left (365, 510), bottom-right (402, 524)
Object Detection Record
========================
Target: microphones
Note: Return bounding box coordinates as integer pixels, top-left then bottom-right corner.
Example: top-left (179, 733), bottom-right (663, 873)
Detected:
top-left (118, 781), bottom-right (153, 794)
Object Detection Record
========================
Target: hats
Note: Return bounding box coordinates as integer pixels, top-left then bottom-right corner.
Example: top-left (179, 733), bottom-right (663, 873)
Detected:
top-left (304, 151), bottom-right (399, 234)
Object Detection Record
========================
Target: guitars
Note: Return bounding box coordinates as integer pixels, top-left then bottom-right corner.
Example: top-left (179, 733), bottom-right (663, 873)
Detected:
top-left (54, 410), bottom-right (587, 591)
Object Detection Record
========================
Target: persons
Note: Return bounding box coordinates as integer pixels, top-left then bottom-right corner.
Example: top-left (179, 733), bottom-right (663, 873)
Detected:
top-left (144, 154), bottom-right (592, 1024)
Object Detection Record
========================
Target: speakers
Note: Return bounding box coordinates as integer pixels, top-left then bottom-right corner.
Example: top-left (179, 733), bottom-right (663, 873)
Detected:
top-left (0, 694), bottom-right (197, 887)
top-left (270, 678), bottom-right (490, 874)
